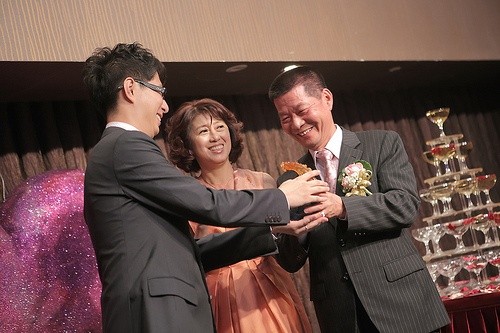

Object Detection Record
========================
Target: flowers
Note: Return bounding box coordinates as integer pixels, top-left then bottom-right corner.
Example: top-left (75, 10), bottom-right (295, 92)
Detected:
top-left (337, 160), bottom-right (374, 196)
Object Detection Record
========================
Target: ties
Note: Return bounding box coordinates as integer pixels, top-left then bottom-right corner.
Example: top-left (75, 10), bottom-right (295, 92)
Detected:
top-left (315, 149), bottom-right (337, 194)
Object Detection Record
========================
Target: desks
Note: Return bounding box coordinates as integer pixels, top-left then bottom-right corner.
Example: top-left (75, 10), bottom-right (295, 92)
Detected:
top-left (434, 291), bottom-right (500, 333)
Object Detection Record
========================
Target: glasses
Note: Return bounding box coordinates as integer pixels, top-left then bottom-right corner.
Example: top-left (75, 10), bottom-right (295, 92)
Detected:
top-left (117, 80), bottom-right (167, 99)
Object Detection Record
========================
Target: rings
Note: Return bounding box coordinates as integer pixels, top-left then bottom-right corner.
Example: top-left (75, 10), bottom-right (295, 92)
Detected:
top-left (305, 225), bottom-right (307, 231)
top-left (321, 211), bottom-right (324, 216)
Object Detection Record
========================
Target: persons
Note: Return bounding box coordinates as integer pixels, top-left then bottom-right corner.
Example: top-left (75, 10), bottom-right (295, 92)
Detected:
top-left (81, 42), bottom-right (330, 333)
top-left (165, 97), bottom-right (313, 333)
top-left (268, 66), bottom-right (451, 333)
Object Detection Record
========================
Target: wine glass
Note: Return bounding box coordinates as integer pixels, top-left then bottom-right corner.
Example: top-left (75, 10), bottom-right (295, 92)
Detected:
top-left (411, 107), bottom-right (500, 301)
